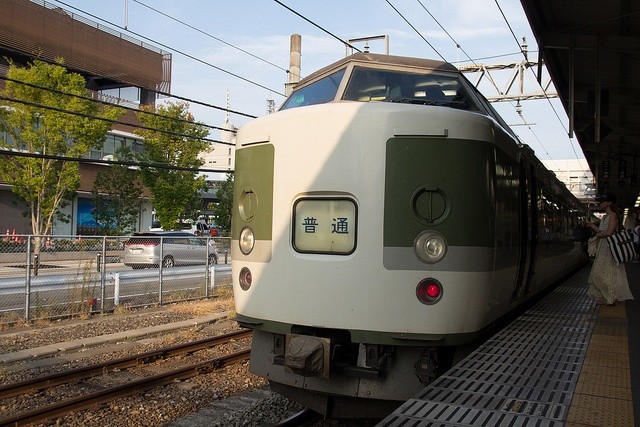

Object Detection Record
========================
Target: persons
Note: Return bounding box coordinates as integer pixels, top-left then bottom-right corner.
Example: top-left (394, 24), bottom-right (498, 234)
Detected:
top-left (632, 218), bottom-right (640, 264)
top-left (209, 238), bottom-right (218, 253)
top-left (582, 194), bottom-right (635, 306)
top-left (615, 208), bottom-right (626, 232)
top-left (627, 229), bottom-right (634, 242)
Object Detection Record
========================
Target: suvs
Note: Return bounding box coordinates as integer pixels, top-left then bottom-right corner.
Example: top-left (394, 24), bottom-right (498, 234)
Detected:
top-left (150, 220), bottom-right (197, 236)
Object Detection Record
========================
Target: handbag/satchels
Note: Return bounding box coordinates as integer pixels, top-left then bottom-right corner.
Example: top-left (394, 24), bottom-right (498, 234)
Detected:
top-left (606, 229), bottom-right (637, 266)
top-left (588, 236), bottom-right (599, 257)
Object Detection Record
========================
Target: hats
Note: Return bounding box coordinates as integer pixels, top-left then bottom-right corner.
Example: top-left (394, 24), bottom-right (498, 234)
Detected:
top-left (595, 192), bottom-right (617, 203)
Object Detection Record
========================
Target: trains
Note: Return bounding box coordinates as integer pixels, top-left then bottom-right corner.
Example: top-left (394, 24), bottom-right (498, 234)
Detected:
top-left (231, 52), bottom-right (600, 420)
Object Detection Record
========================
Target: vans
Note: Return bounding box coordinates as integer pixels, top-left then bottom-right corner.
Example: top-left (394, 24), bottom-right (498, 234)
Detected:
top-left (124, 231), bottom-right (219, 269)
